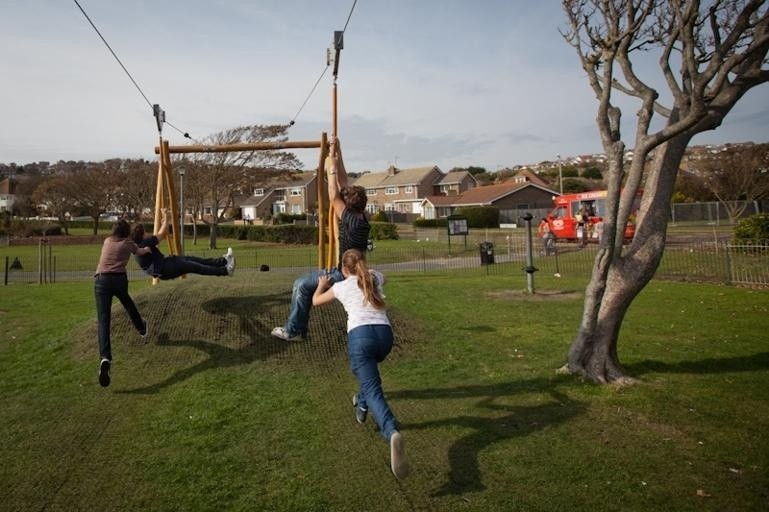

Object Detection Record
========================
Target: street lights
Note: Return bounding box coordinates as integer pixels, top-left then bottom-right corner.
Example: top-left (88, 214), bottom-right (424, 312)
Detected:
top-left (178, 162), bottom-right (188, 255)
top-left (556, 152), bottom-right (564, 193)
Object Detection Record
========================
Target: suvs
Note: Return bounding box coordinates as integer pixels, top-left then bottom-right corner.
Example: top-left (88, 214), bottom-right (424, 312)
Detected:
top-left (103, 216), bottom-right (122, 222)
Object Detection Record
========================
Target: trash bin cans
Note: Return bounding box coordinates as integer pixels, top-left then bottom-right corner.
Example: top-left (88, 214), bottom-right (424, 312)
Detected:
top-left (480, 242), bottom-right (494, 265)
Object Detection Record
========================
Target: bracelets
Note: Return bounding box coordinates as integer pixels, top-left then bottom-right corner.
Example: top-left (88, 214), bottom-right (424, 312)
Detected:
top-left (327, 171), bottom-right (336, 176)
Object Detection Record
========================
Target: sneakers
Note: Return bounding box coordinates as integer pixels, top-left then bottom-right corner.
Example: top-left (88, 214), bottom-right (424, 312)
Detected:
top-left (139, 318), bottom-right (149, 339)
top-left (388, 430), bottom-right (411, 482)
top-left (222, 246), bottom-right (233, 268)
top-left (270, 325), bottom-right (306, 343)
top-left (226, 258), bottom-right (237, 279)
top-left (98, 356), bottom-right (112, 388)
top-left (352, 393), bottom-right (368, 426)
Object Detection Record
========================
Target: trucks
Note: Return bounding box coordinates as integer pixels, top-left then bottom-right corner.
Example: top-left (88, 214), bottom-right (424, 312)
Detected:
top-left (540, 187), bottom-right (646, 243)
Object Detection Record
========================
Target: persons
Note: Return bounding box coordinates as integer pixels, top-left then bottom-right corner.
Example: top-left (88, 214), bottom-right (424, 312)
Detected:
top-left (269, 136), bottom-right (369, 346)
top-left (128, 207), bottom-right (237, 280)
top-left (538, 218), bottom-right (556, 256)
top-left (94, 219), bottom-right (149, 387)
top-left (310, 248), bottom-right (409, 481)
top-left (573, 204), bottom-right (606, 251)
